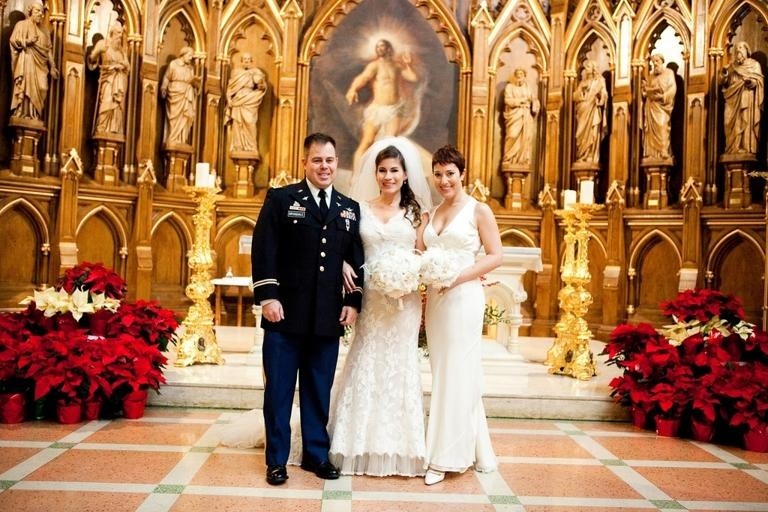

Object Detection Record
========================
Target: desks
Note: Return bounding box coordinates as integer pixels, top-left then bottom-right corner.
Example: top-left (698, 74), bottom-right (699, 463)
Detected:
top-left (211, 278), bottom-right (255, 326)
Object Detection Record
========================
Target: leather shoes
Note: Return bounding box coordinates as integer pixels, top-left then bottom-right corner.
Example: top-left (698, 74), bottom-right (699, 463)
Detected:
top-left (265, 464), bottom-right (287, 485)
top-left (424, 468), bottom-right (446, 486)
top-left (301, 461), bottom-right (339, 479)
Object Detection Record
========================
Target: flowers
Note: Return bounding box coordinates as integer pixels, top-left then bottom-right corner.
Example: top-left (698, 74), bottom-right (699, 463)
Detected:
top-left (604, 288), bottom-right (767, 435)
top-left (0, 261), bottom-right (184, 403)
top-left (361, 248), bottom-right (421, 310)
top-left (421, 246), bottom-right (460, 295)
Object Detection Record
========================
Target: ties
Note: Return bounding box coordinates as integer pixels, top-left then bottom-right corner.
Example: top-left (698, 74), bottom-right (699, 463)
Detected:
top-left (317, 188), bottom-right (329, 219)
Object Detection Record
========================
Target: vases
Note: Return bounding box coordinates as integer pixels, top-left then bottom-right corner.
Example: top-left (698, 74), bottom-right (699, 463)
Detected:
top-left (84, 402), bottom-right (101, 420)
top-left (119, 390), bottom-right (147, 420)
top-left (1, 394), bottom-right (26, 424)
top-left (687, 417), bottom-right (716, 444)
top-left (55, 403), bottom-right (81, 423)
top-left (741, 429), bottom-right (766, 452)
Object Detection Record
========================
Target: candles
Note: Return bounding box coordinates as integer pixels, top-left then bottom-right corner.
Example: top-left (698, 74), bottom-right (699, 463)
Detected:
top-left (579, 180), bottom-right (594, 204)
top-left (196, 163), bottom-right (208, 188)
top-left (207, 176), bottom-right (214, 188)
top-left (564, 189), bottom-right (577, 211)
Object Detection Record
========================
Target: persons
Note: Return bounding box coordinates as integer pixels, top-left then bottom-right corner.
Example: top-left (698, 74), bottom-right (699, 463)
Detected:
top-left (251, 133), bottom-right (365, 483)
top-left (503, 68), bottom-right (541, 167)
top-left (161, 45), bottom-right (198, 146)
top-left (89, 23), bottom-right (130, 135)
top-left (571, 60), bottom-right (609, 166)
top-left (223, 52), bottom-right (268, 160)
top-left (327, 136), bottom-right (432, 479)
top-left (9, 4), bottom-right (56, 120)
top-left (422, 146), bottom-right (503, 486)
top-left (346, 40), bottom-right (418, 185)
top-left (717, 42), bottom-right (764, 154)
top-left (640, 52), bottom-right (677, 159)
